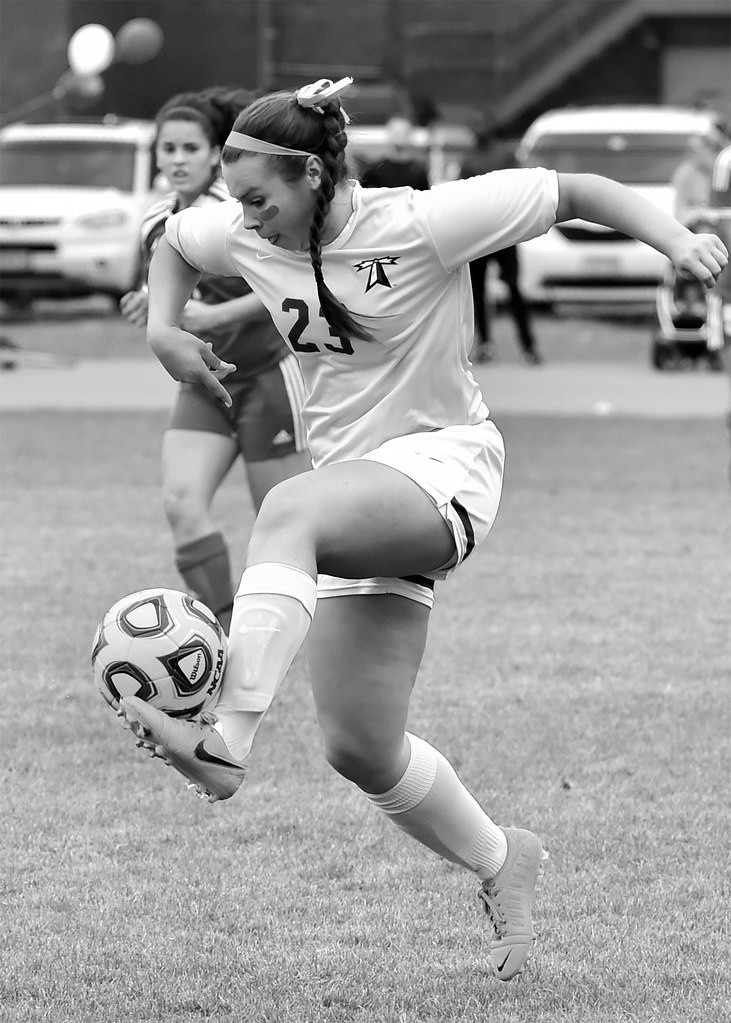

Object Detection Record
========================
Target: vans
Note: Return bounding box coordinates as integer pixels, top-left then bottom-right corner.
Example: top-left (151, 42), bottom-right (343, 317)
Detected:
top-left (514, 103), bottom-right (731, 319)
top-left (341, 124), bottom-right (475, 201)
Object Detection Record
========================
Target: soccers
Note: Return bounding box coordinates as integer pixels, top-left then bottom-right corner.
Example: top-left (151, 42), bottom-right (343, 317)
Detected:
top-left (90, 588), bottom-right (230, 720)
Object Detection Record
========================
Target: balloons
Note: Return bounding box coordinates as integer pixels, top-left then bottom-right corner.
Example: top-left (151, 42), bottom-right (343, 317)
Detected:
top-left (60, 72), bottom-right (106, 109)
top-left (114, 18), bottom-right (164, 63)
top-left (68, 24), bottom-right (115, 80)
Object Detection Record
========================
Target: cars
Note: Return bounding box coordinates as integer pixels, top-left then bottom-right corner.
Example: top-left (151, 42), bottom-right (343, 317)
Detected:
top-left (0, 113), bottom-right (157, 313)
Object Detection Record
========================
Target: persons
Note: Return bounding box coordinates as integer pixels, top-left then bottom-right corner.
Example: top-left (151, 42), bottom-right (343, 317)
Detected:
top-left (675, 121), bottom-right (731, 339)
top-left (118, 77), bottom-right (731, 981)
top-left (360, 119), bottom-right (431, 191)
top-left (458, 124), bottom-right (540, 360)
top-left (120, 88), bottom-right (314, 635)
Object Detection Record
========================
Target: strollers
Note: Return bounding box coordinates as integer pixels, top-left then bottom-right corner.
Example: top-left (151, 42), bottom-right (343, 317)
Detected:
top-left (646, 251), bottom-right (729, 370)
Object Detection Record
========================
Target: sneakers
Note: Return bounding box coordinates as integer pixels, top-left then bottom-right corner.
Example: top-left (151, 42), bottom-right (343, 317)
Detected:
top-left (118, 695), bottom-right (248, 804)
top-left (475, 826), bottom-right (549, 982)
